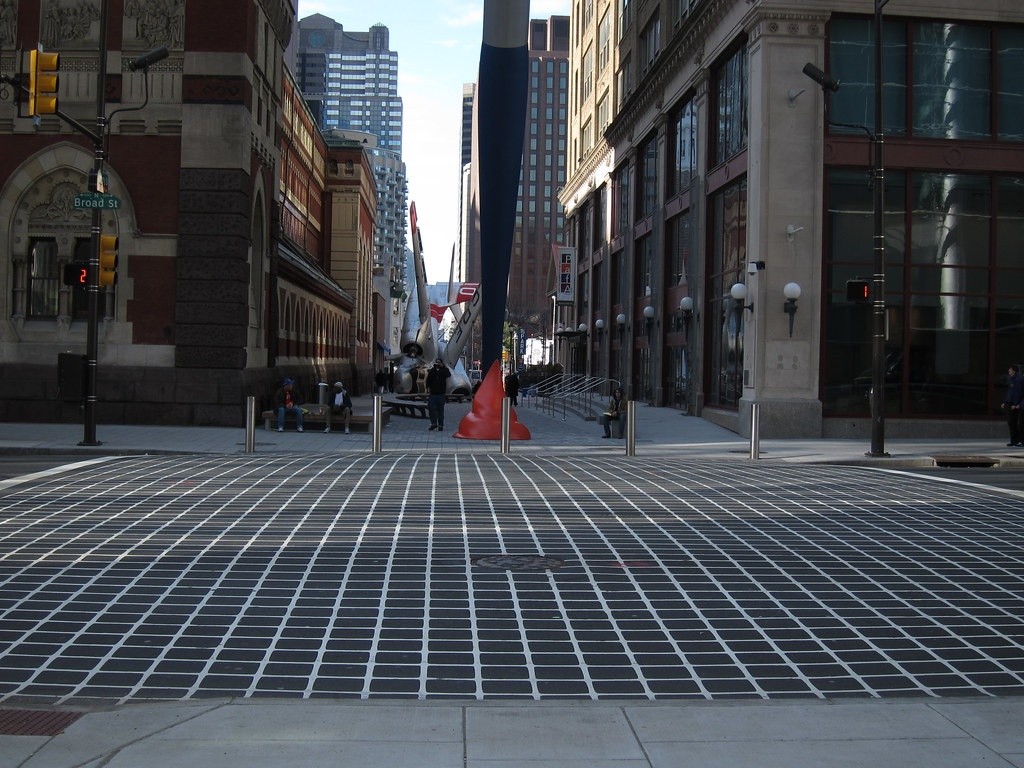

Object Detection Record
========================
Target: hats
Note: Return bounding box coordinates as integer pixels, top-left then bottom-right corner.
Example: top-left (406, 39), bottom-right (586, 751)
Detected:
top-left (283, 379), bottom-right (294, 386)
top-left (335, 382), bottom-right (343, 388)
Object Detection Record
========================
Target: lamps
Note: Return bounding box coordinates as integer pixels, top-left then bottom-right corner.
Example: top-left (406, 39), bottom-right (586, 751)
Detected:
top-left (680, 297), bottom-right (700, 342)
top-left (783, 282), bottom-right (801, 339)
top-left (731, 283), bottom-right (754, 337)
top-left (616, 314), bottom-right (625, 346)
top-left (557, 319), bottom-right (604, 351)
top-left (644, 306), bottom-right (654, 344)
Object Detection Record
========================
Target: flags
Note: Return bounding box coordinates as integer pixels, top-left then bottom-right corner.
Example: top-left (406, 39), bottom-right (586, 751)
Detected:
top-left (456, 281), bottom-right (481, 305)
top-left (430, 303), bottom-right (448, 322)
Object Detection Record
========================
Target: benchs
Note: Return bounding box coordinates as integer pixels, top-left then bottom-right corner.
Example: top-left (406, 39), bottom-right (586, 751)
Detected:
top-left (379, 391), bottom-right (464, 419)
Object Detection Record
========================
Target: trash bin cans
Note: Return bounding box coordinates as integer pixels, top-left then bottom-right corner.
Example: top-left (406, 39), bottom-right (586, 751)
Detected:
top-left (319, 383), bottom-right (328, 407)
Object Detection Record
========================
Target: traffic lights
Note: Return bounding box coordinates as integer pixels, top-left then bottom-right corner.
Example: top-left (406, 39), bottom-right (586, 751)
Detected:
top-left (63, 261), bottom-right (90, 286)
top-left (29, 50), bottom-right (59, 118)
top-left (845, 279), bottom-right (871, 300)
top-left (100, 233), bottom-right (120, 288)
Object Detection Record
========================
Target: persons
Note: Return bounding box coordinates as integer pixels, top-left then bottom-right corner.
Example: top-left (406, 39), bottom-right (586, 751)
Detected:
top-left (425, 359), bottom-right (451, 431)
top-left (601, 387), bottom-right (627, 440)
top-left (272, 379), bottom-right (304, 433)
top-left (1001, 365), bottom-right (1024, 447)
top-left (506, 373), bottom-right (519, 408)
top-left (323, 381), bottom-right (353, 434)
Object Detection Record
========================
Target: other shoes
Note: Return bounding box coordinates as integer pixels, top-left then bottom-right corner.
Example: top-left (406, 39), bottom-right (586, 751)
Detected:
top-left (429, 425), bottom-right (443, 431)
top-left (297, 427), bottom-right (304, 433)
top-left (345, 426), bottom-right (350, 434)
top-left (278, 428), bottom-right (284, 432)
top-left (1007, 443), bottom-right (1024, 449)
top-left (323, 427), bottom-right (330, 433)
